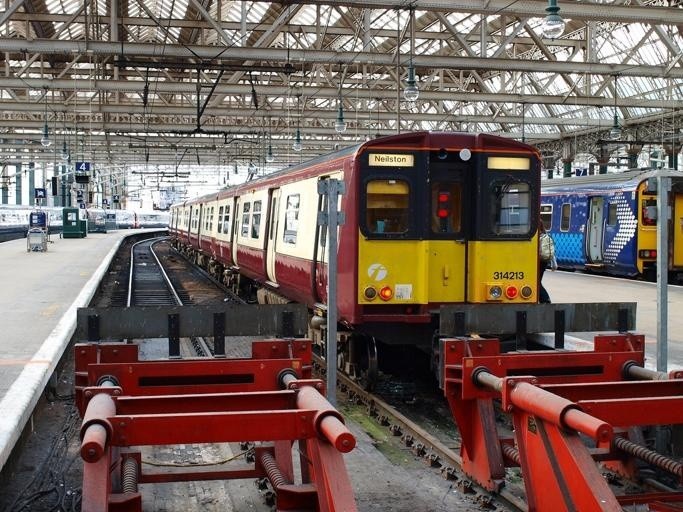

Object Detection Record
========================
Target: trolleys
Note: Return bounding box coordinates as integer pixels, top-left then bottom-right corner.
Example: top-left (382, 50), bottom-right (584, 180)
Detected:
top-left (27, 211), bottom-right (54, 252)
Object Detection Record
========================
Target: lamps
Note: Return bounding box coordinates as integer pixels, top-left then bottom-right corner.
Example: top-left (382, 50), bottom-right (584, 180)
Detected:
top-left (266, 145), bottom-right (275, 162)
top-left (540, 2), bottom-right (569, 42)
top-left (219, 147), bottom-right (263, 186)
top-left (292, 129), bottom-right (302, 153)
top-left (60, 141), bottom-right (69, 158)
top-left (40, 120), bottom-right (52, 147)
top-left (402, 65), bottom-right (421, 104)
top-left (608, 112), bottom-right (624, 145)
top-left (335, 105), bottom-right (348, 134)
top-left (66, 160), bottom-right (72, 170)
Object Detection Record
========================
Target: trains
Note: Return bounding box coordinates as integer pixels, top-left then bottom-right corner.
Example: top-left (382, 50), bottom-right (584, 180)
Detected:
top-left (170, 130), bottom-right (540, 393)
top-left (541, 166), bottom-right (683, 277)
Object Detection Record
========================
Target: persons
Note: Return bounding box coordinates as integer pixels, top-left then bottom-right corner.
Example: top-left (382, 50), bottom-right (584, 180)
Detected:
top-left (539, 219), bottom-right (556, 304)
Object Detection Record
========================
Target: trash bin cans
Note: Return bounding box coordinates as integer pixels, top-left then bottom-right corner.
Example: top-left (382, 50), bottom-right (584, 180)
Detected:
top-left (104, 214), bottom-right (116, 229)
top-left (62, 207), bottom-right (87, 239)
